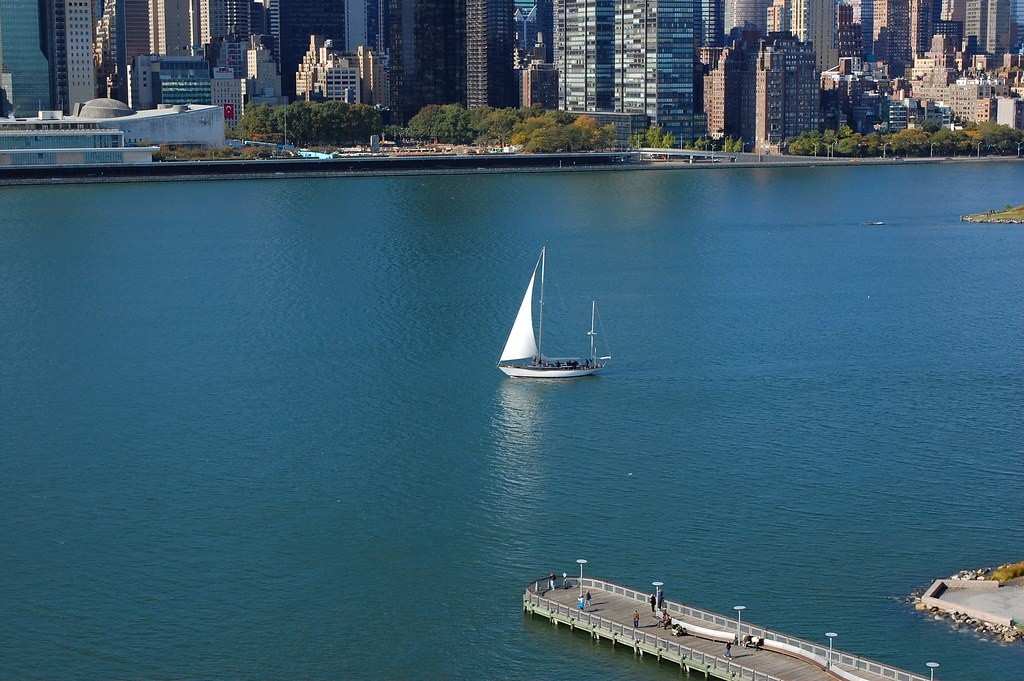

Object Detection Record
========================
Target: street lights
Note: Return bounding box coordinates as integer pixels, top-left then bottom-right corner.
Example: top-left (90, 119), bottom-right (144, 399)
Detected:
top-left (825, 632), bottom-right (837, 668)
top-left (832, 142), bottom-right (834, 158)
top-left (977, 142), bottom-right (982, 158)
top-left (827, 145), bottom-right (831, 159)
top-left (926, 662), bottom-right (940, 681)
top-left (652, 582), bottom-right (664, 613)
top-left (733, 606), bottom-right (747, 646)
top-left (725, 135), bottom-right (730, 152)
top-left (815, 145), bottom-right (817, 158)
top-left (883, 143), bottom-right (888, 159)
top-left (577, 559), bottom-right (589, 597)
top-left (931, 142), bottom-right (936, 157)
top-left (1015, 141), bottom-right (1023, 157)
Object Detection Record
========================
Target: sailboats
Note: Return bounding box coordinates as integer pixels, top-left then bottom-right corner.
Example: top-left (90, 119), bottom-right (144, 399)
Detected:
top-left (495, 248), bottom-right (612, 378)
